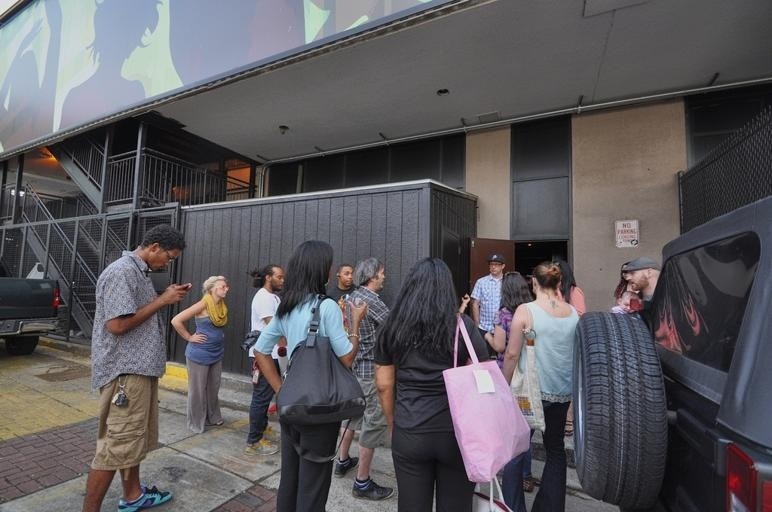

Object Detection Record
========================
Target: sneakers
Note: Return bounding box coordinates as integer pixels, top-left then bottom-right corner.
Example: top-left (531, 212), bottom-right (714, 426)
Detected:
top-left (244, 438), bottom-right (280, 456)
top-left (118, 484), bottom-right (172, 512)
top-left (263, 425), bottom-right (281, 442)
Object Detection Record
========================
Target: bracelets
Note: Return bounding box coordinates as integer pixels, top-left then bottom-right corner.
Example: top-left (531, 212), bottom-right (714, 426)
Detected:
top-left (347, 334), bottom-right (359, 339)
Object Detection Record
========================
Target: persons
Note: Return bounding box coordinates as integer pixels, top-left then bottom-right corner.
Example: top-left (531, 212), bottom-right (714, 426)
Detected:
top-left (553, 257), bottom-right (585, 436)
top-left (253, 239), bottom-right (367, 512)
top-left (484, 272), bottom-right (533, 371)
top-left (242, 265), bottom-right (285, 456)
top-left (470, 254), bottom-right (505, 338)
top-left (341, 257), bottom-right (394, 501)
top-left (170, 275), bottom-right (229, 433)
top-left (330, 263), bottom-right (358, 312)
top-left (612, 261), bottom-right (642, 298)
top-left (622, 257), bottom-right (661, 314)
top-left (79, 224), bottom-right (192, 512)
top-left (609, 291), bottom-right (642, 313)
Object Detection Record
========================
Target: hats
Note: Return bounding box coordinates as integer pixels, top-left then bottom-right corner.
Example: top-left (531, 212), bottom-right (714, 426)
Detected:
top-left (622, 256), bottom-right (662, 272)
top-left (487, 252), bottom-right (507, 264)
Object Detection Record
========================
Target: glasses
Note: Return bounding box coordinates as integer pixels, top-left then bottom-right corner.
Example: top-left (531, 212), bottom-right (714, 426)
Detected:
top-left (166, 250), bottom-right (176, 263)
top-left (216, 285), bottom-right (230, 289)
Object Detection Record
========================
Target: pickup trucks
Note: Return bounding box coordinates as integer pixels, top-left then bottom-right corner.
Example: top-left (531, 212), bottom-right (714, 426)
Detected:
top-left (0, 257), bottom-right (61, 356)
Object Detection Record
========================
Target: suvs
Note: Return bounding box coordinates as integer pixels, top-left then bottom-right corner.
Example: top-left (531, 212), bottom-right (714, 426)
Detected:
top-left (571, 196), bottom-right (771, 512)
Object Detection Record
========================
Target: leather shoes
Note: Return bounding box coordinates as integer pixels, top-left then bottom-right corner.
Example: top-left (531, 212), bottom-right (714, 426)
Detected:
top-left (216, 420), bottom-right (224, 426)
top-left (334, 456), bottom-right (359, 477)
top-left (522, 479), bottom-right (536, 492)
top-left (353, 479), bottom-right (394, 500)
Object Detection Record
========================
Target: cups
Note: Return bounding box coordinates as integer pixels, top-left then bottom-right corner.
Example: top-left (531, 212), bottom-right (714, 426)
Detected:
top-left (350, 298), bottom-right (363, 322)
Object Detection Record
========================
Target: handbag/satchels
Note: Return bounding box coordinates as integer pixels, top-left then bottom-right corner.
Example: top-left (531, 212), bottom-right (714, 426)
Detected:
top-left (276, 296), bottom-right (366, 425)
top-left (442, 311), bottom-right (531, 483)
top-left (510, 304), bottom-right (547, 434)
top-left (472, 475), bottom-right (514, 512)
